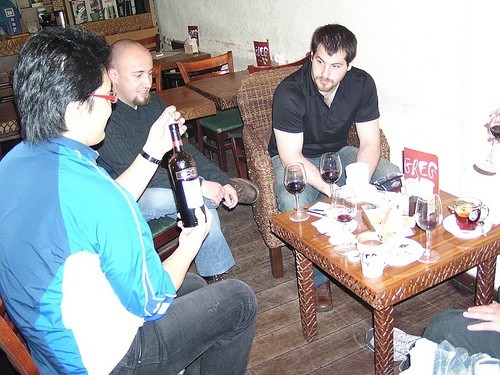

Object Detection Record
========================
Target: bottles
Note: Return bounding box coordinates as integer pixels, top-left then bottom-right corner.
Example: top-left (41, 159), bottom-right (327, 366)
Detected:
top-left (167, 123), bottom-right (206, 228)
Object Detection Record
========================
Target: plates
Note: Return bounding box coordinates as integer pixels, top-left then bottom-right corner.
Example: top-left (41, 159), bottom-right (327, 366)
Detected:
top-left (339, 184), bottom-right (378, 203)
top-left (442, 214), bottom-right (492, 240)
top-left (382, 238), bottom-right (424, 268)
top-left (316, 217), bottom-right (358, 237)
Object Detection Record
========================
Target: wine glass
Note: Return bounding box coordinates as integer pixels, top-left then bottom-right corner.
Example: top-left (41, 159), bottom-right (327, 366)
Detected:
top-left (330, 188), bottom-right (357, 255)
top-left (319, 152), bottom-right (342, 210)
top-left (283, 162), bottom-right (310, 222)
top-left (473, 111), bottom-right (500, 175)
top-left (414, 193), bottom-right (444, 264)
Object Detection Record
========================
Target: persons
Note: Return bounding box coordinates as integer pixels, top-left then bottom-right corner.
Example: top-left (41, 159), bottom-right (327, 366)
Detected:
top-left (364, 303), bottom-right (500, 375)
top-left (0, 26), bottom-right (257, 375)
top-left (266, 24), bottom-right (403, 313)
top-left (99, 38), bottom-right (259, 284)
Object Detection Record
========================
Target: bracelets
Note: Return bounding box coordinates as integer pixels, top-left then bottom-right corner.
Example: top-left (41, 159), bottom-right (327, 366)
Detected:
top-left (139, 149), bottom-right (162, 166)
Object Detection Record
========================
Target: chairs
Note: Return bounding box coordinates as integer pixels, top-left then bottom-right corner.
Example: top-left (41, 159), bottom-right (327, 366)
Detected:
top-left (0, 28), bottom-right (390, 375)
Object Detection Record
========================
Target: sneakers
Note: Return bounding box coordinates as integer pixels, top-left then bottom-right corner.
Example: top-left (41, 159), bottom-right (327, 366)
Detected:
top-left (198, 272), bottom-right (224, 285)
top-left (365, 327), bottom-right (421, 362)
top-left (230, 177), bottom-right (259, 203)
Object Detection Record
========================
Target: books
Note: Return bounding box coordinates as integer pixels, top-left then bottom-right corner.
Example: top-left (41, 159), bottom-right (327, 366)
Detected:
top-left (71, 0), bottom-right (151, 24)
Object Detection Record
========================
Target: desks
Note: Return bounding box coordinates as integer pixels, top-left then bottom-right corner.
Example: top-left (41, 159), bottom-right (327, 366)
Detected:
top-left (148, 48), bottom-right (211, 87)
top-left (150, 87), bottom-right (216, 157)
top-left (188, 66), bottom-right (249, 161)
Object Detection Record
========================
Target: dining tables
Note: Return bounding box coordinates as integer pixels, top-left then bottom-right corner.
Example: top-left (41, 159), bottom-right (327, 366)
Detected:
top-left (269, 179), bottom-right (500, 375)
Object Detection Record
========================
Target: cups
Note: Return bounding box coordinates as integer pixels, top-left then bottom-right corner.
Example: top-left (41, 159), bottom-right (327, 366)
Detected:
top-left (356, 232), bottom-right (387, 279)
top-left (346, 162), bottom-right (370, 190)
top-left (38, 10), bottom-right (67, 29)
top-left (453, 196), bottom-right (490, 231)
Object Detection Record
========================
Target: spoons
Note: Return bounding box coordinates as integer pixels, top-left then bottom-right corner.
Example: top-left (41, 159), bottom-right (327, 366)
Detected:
top-left (446, 206), bottom-right (457, 215)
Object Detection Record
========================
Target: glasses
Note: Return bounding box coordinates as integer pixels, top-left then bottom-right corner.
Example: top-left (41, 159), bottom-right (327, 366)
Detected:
top-left (88, 92), bottom-right (118, 103)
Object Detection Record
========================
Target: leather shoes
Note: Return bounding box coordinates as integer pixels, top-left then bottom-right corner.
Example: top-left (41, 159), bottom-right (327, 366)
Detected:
top-left (314, 279), bottom-right (333, 312)
